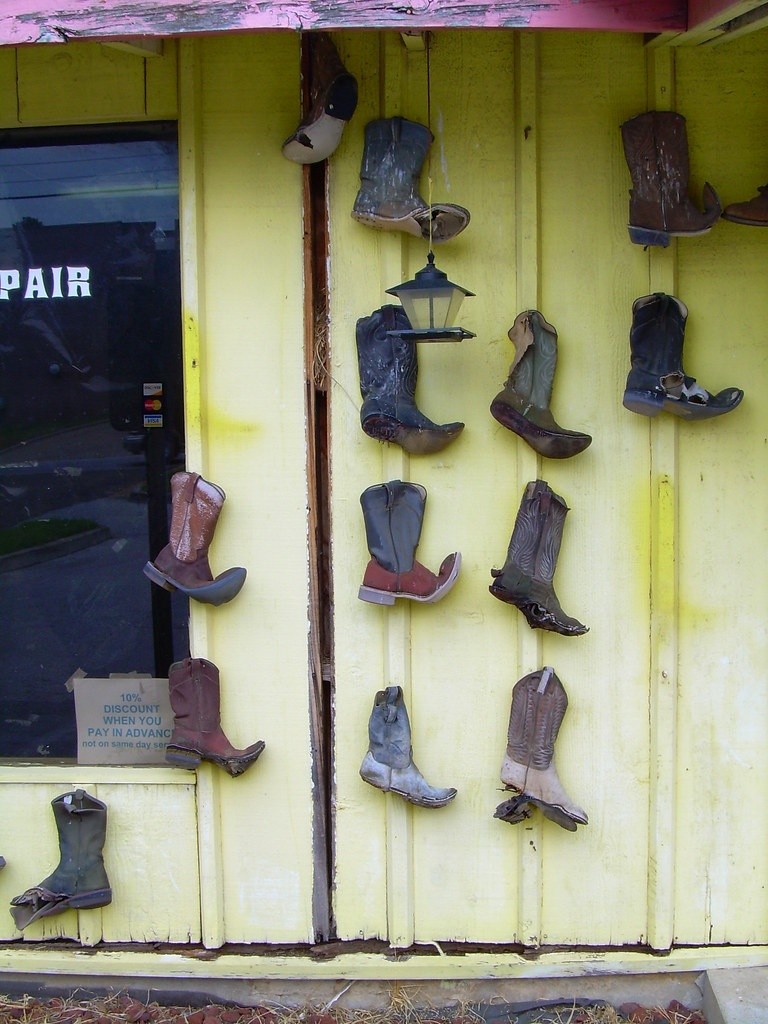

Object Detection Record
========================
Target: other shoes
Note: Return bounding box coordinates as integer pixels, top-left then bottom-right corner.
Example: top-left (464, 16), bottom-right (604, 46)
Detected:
top-left (721, 182), bottom-right (768, 227)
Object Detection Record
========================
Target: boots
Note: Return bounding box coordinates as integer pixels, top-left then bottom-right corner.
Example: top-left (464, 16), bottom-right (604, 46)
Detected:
top-left (281, 31), bottom-right (358, 164)
top-left (619, 110), bottom-right (723, 252)
top-left (10, 790), bottom-right (113, 932)
top-left (355, 304), bottom-right (465, 455)
top-left (359, 685), bottom-right (458, 809)
top-left (489, 478), bottom-right (590, 636)
top-left (166, 657), bottom-right (266, 778)
top-left (489, 310), bottom-right (592, 459)
top-left (492, 667), bottom-right (589, 832)
top-left (351, 115), bottom-right (471, 242)
top-left (622, 292), bottom-right (744, 421)
top-left (358, 479), bottom-right (462, 606)
top-left (143, 470), bottom-right (246, 606)
